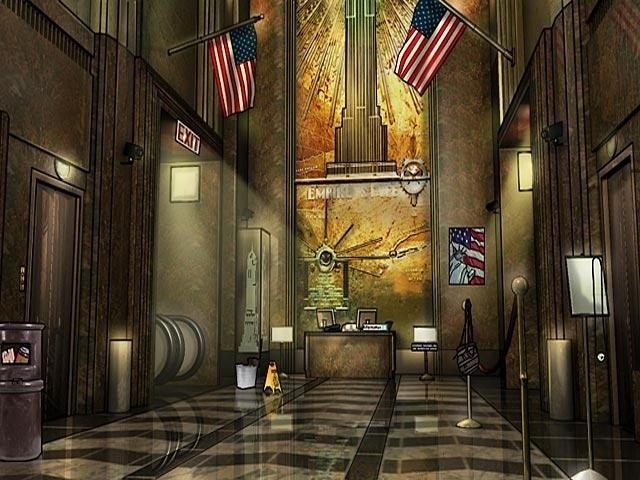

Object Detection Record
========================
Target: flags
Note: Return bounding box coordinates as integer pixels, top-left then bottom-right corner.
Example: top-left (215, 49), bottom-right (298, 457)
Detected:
top-left (209, 22), bottom-right (257, 119)
top-left (393, 1), bottom-right (466, 96)
